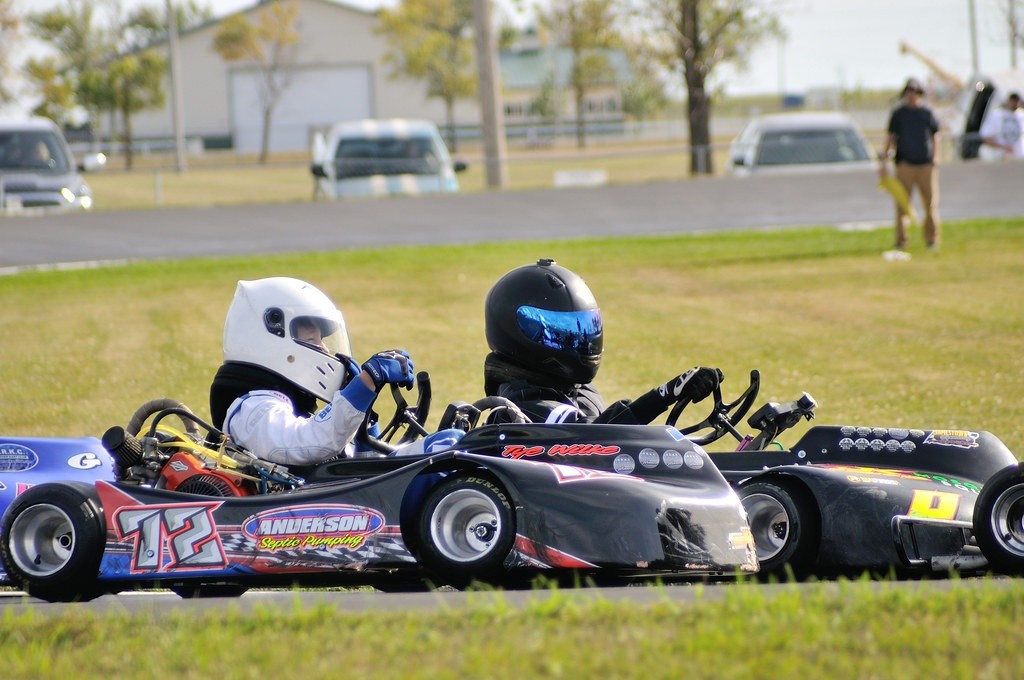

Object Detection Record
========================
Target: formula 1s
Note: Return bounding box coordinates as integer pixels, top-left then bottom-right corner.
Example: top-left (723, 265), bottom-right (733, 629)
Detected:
top-left (668, 363), bottom-right (1024, 588)
top-left (2, 371), bottom-right (759, 601)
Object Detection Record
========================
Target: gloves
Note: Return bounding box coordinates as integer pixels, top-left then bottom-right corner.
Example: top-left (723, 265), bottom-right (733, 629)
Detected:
top-left (337, 352), bottom-right (360, 386)
top-left (364, 350), bottom-right (413, 394)
top-left (659, 364), bottom-right (721, 405)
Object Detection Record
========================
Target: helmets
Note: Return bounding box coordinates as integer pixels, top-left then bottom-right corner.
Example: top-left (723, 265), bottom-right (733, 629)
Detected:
top-left (484, 260), bottom-right (607, 389)
top-left (221, 275), bottom-right (354, 401)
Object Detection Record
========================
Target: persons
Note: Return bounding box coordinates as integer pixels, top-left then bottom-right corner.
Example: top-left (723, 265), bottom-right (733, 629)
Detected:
top-left (878, 77), bottom-right (942, 260)
top-left (484, 261), bottom-right (721, 424)
top-left (979, 90), bottom-right (1024, 160)
top-left (211, 274), bottom-right (469, 455)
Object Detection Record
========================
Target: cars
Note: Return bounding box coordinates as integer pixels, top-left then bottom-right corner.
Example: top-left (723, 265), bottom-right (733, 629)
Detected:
top-left (723, 110), bottom-right (884, 179)
top-left (307, 119), bottom-right (468, 207)
top-left (0, 115), bottom-right (106, 223)
